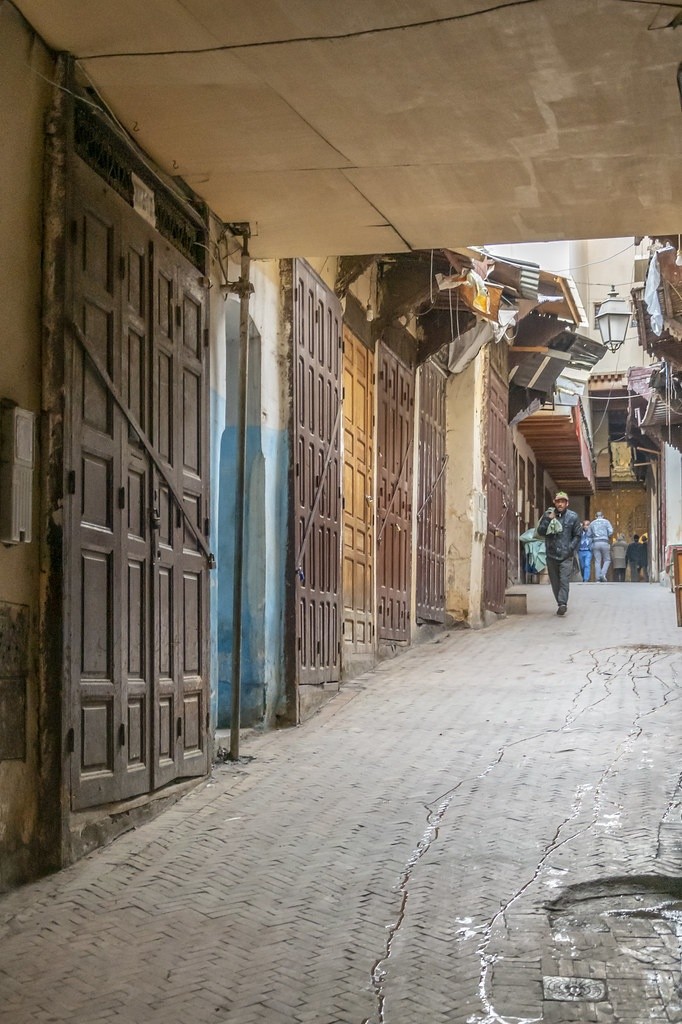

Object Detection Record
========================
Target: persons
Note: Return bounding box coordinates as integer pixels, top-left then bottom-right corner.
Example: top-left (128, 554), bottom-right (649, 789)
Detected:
top-left (586, 511), bottom-right (613, 583)
top-left (625, 535), bottom-right (643, 582)
top-left (612, 536), bottom-right (628, 582)
top-left (537, 491), bottom-right (582, 615)
top-left (578, 520), bottom-right (592, 583)
top-left (642, 536), bottom-right (650, 582)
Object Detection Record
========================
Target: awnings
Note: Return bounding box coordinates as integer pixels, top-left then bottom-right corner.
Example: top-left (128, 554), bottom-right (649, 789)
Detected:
top-left (376, 244), bottom-right (589, 326)
top-left (509, 317), bottom-right (609, 406)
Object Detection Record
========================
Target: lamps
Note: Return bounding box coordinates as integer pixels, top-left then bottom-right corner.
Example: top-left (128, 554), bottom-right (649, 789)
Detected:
top-left (595, 285), bottom-right (634, 353)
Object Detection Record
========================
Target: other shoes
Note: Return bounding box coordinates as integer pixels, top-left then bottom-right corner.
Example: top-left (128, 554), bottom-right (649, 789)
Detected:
top-left (600, 574), bottom-right (609, 582)
top-left (595, 578), bottom-right (600, 582)
top-left (558, 604), bottom-right (567, 615)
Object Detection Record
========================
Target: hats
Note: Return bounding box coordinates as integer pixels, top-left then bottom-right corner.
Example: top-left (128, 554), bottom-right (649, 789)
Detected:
top-left (554, 492), bottom-right (569, 502)
